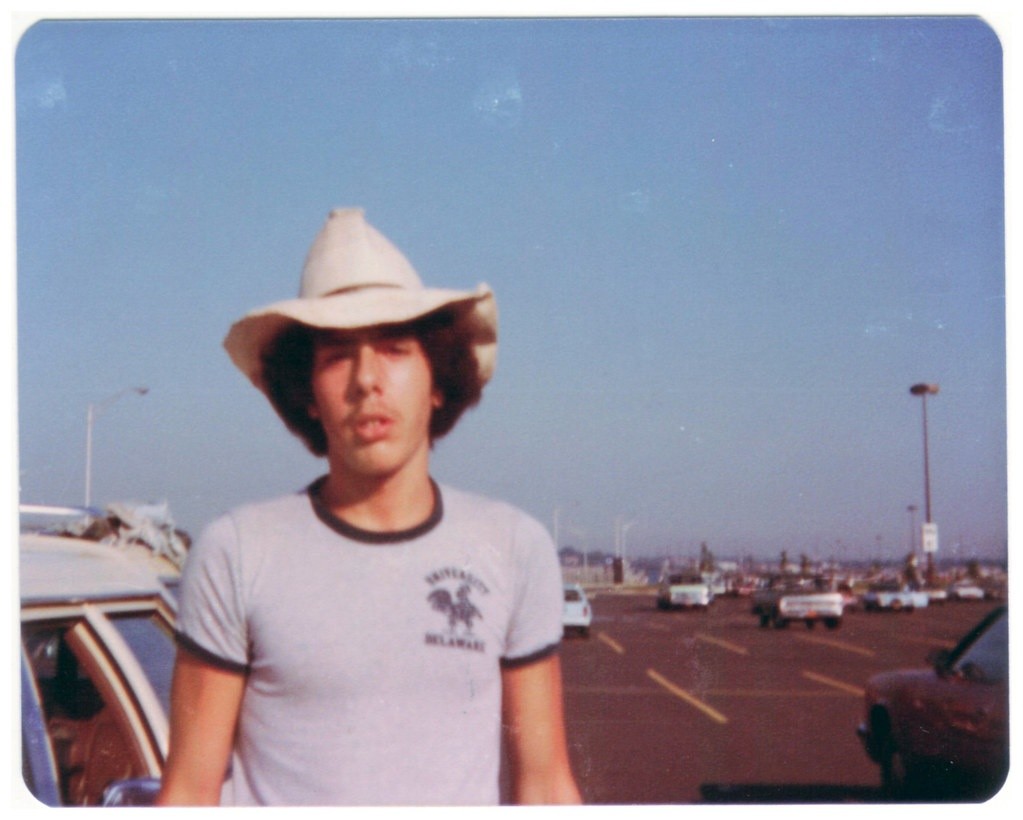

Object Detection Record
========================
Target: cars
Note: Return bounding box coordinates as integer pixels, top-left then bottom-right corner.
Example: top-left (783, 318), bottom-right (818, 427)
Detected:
top-left (859, 594), bottom-right (1009, 803)
top-left (563, 581), bottom-right (595, 639)
top-left (657, 571), bottom-right (711, 612)
top-left (703, 563), bottom-right (1008, 634)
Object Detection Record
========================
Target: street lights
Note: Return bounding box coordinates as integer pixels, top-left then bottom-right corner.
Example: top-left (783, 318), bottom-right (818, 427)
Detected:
top-left (911, 382), bottom-right (939, 585)
top-left (81, 383), bottom-right (148, 508)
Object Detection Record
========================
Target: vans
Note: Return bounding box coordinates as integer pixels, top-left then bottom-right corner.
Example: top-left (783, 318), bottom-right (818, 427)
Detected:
top-left (20, 500), bottom-right (227, 809)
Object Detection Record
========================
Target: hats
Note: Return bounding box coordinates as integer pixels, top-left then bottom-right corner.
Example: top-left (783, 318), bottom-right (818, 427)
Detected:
top-left (224, 210), bottom-right (499, 406)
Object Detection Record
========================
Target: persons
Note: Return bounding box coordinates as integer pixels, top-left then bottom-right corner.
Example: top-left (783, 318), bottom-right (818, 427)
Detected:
top-left (106, 516), bottom-right (122, 547)
top-left (153, 207), bottom-right (585, 805)
